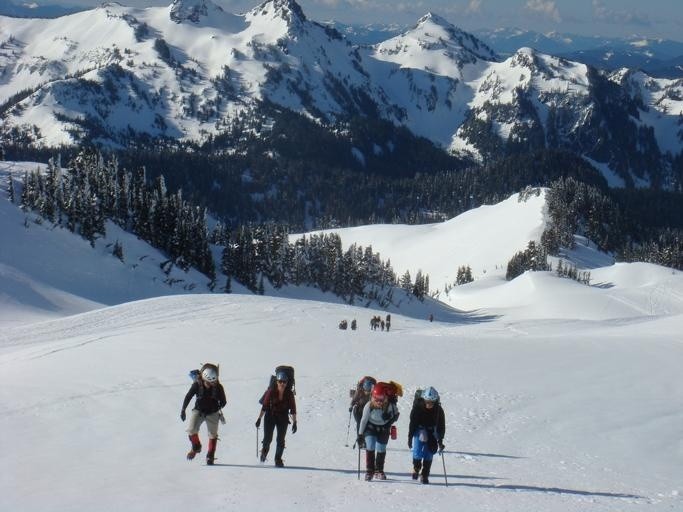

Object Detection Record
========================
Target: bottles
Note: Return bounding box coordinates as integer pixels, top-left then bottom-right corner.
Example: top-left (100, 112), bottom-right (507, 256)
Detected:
top-left (218, 412), bottom-right (227, 425)
top-left (390, 425), bottom-right (397, 440)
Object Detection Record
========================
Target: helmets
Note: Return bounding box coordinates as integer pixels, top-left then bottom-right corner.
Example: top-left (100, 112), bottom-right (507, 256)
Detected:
top-left (420, 385), bottom-right (440, 404)
top-left (201, 367), bottom-right (218, 383)
top-left (275, 371), bottom-right (289, 382)
top-left (371, 382), bottom-right (388, 402)
top-left (363, 379), bottom-right (373, 393)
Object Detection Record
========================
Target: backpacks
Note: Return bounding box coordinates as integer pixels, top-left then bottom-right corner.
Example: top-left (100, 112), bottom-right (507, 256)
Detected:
top-left (411, 389), bottom-right (441, 411)
top-left (259, 365), bottom-right (296, 411)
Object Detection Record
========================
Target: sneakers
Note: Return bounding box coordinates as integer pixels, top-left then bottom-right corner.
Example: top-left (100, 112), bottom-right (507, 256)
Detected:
top-left (186, 446), bottom-right (203, 461)
top-left (275, 457), bottom-right (283, 467)
top-left (261, 449), bottom-right (267, 462)
top-left (420, 475), bottom-right (429, 484)
top-left (373, 471), bottom-right (386, 480)
top-left (365, 473), bottom-right (373, 481)
top-left (206, 452), bottom-right (215, 465)
top-left (412, 471), bottom-right (419, 479)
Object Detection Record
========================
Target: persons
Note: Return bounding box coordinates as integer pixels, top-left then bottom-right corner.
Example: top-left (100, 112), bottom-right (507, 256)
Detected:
top-left (347, 376), bottom-right (377, 451)
top-left (356, 383), bottom-right (400, 482)
top-left (338, 314), bottom-right (390, 333)
top-left (180, 366), bottom-right (226, 465)
top-left (406, 387), bottom-right (445, 485)
top-left (255, 370), bottom-right (298, 467)
top-left (428, 314), bottom-right (433, 322)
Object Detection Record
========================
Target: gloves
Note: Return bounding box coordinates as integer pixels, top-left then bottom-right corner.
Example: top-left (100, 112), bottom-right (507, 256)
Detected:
top-left (357, 434), bottom-right (366, 448)
top-left (181, 410), bottom-right (187, 422)
top-left (292, 421), bottom-right (297, 433)
top-left (437, 440), bottom-right (445, 451)
top-left (408, 437), bottom-right (412, 449)
top-left (256, 418), bottom-right (261, 427)
